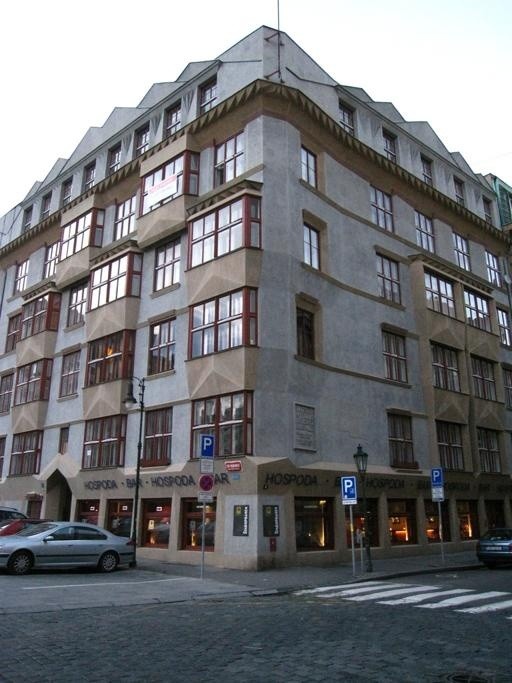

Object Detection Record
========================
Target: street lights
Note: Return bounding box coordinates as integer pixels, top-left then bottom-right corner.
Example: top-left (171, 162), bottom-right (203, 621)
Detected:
top-left (352, 442), bottom-right (373, 573)
top-left (120, 375), bottom-right (146, 568)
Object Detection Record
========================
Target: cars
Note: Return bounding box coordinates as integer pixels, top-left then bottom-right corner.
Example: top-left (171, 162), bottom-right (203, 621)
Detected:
top-left (0, 505), bottom-right (137, 576)
top-left (476, 527), bottom-right (512, 570)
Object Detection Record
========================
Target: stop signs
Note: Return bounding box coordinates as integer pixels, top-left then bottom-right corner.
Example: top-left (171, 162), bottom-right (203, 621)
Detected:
top-left (198, 473), bottom-right (214, 492)
